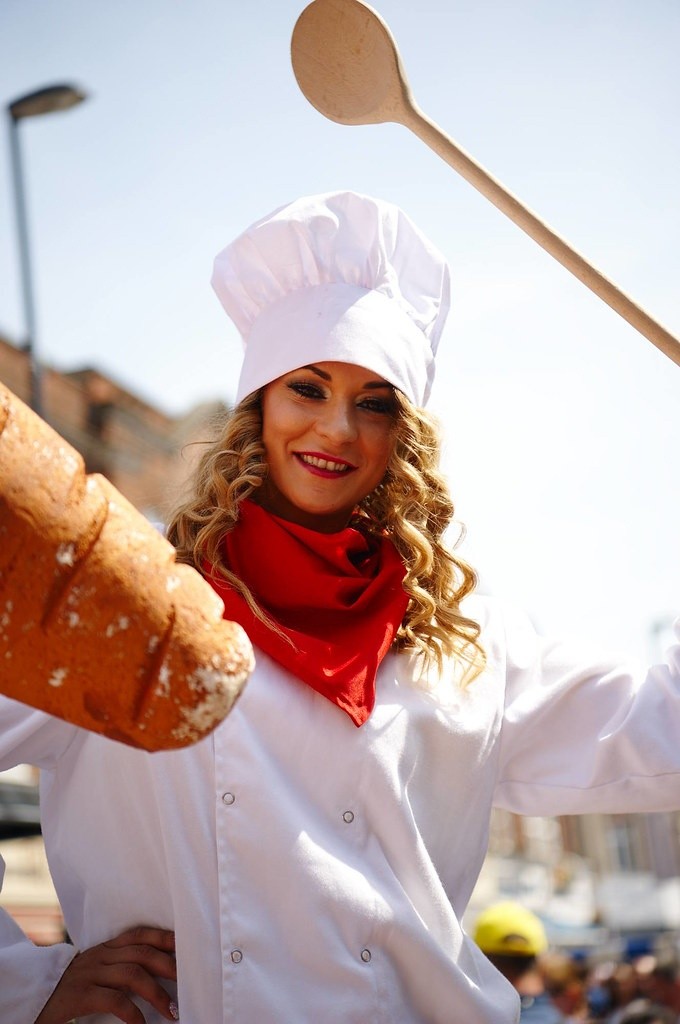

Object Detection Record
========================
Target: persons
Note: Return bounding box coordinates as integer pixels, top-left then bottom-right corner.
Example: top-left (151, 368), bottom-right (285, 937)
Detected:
top-left (0, 190), bottom-right (680, 1024)
top-left (472, 903), bottom-right (680, 1024)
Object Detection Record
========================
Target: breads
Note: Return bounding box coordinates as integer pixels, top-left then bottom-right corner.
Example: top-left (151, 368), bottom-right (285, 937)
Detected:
top-left (0, 381), bottom-right (256, 755)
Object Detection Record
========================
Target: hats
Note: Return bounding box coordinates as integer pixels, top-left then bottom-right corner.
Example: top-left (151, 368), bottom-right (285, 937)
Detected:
top-left (210, 191), bottom-right (451, 414)
top-left (473, 901), bottom-right (548, 956)
